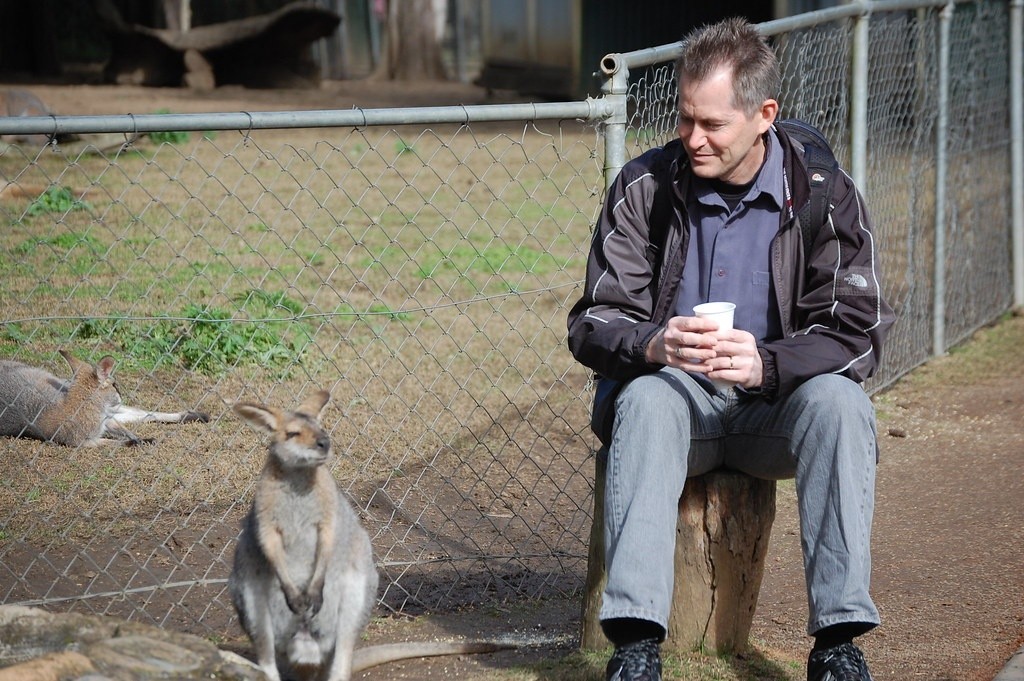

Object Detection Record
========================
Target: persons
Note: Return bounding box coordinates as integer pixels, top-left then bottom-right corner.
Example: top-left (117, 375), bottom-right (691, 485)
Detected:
top-left (566, 18), bottom-right (896, 681)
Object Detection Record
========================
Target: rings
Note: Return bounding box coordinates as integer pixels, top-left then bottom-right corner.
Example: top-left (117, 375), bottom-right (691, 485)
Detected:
top-left (728, 355), bottom-right (733, 369)
top-left (675, 347), bottom-right (683, 359)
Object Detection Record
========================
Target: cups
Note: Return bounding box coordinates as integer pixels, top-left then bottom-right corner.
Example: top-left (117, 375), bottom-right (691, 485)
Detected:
top-left (692, 301), bottom-right (736, 334)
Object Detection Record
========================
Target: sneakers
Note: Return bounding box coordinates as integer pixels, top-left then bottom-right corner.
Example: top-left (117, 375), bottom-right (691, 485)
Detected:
top-left (807, 642), bottom-right (871, 681)
top-left (606, 638), bottom-right (663, 681)
